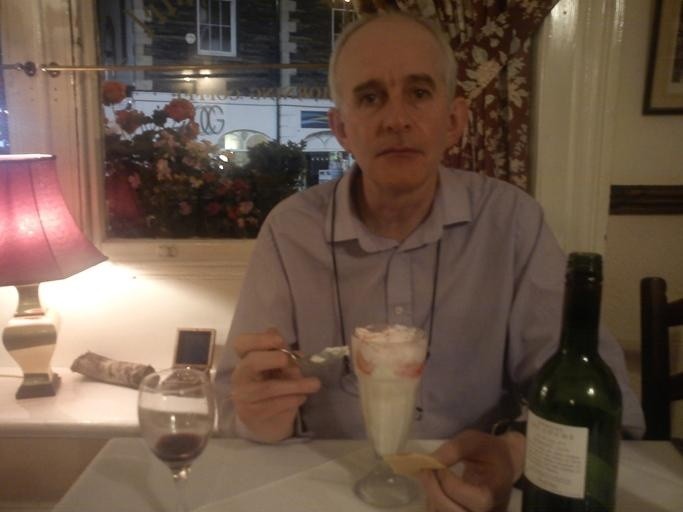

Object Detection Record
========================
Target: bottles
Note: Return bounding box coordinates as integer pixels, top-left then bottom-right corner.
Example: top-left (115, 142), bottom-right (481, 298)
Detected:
top-left (521, 251), bottom-right (623, 512)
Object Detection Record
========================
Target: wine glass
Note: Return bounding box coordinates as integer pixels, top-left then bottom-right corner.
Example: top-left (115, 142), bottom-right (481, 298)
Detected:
top-left (137, 366), bottom-right (215, 512)
top-left (348, 320), bottom-right (431, 508)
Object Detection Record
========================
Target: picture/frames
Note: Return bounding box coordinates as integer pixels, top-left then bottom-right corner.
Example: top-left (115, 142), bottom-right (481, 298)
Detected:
top-left (639, 1), bottom-right (681, 117)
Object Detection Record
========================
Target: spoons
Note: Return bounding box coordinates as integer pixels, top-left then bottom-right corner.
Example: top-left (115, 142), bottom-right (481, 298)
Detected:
top-left (277, 343), bottom-right (351, 372)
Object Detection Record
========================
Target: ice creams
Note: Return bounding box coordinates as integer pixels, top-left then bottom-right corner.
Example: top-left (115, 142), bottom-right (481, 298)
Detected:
top-left (351, 323), bottom-right (430, 457)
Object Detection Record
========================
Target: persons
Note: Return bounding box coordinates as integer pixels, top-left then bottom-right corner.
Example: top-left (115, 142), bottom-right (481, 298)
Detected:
top-left (207, 11), bottom-right (647, 512)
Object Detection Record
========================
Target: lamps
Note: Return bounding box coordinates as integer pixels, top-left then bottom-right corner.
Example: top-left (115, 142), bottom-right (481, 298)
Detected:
top-left (0, 153), bottom-right (110, 399)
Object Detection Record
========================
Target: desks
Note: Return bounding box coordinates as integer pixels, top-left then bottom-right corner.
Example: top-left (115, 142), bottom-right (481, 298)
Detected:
top-left (0, 371), bottom-right (166, 511)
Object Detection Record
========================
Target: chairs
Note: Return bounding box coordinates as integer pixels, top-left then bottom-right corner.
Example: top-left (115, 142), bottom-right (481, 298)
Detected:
top-left (639, 276), bottom-right (683, 442)
